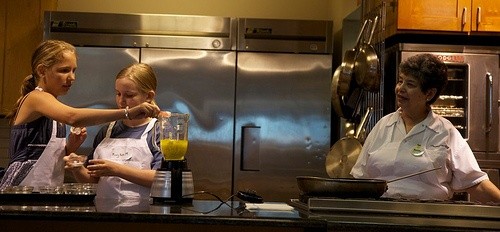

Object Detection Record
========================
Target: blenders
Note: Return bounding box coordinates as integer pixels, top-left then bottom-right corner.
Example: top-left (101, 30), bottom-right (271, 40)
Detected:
top-left (150, 113), bottom-right (194, 207)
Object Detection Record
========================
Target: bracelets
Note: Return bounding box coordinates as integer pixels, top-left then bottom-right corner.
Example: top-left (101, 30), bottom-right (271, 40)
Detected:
top-left (124, 105), bottom-right (131, 120)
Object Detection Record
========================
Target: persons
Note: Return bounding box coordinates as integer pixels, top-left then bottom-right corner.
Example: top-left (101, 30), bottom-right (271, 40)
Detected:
top-left (0, 40), bottom-right (160, 193)
top-left (348, 54), bottom-right (500, 205)
top-left (62, 64), bottom-right (165, 200)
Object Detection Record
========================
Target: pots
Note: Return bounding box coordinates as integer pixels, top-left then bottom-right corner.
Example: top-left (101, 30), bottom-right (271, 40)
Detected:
top-left (296, 177), bottom-right (388, 198)
top-left (326, 15), bottom-right (381, 178)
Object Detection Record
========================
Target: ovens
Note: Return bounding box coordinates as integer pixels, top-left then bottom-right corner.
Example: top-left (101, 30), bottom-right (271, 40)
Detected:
top-left (384, 35), bottom-right (500, 168)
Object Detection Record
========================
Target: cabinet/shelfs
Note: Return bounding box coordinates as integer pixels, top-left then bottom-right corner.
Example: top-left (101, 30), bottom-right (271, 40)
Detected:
top-left (384, 0), bottom-right (500, 42)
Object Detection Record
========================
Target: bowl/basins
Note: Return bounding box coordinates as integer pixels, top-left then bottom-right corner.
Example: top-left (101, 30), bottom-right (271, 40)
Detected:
top-left (69, 155), bottom-right (87, 167)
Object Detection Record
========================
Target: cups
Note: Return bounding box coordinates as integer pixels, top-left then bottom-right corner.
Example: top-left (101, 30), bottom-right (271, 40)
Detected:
top-left (0, 206), bottom-right (95, 212)
top-left (0, 183), bottom-right (96, 194)
